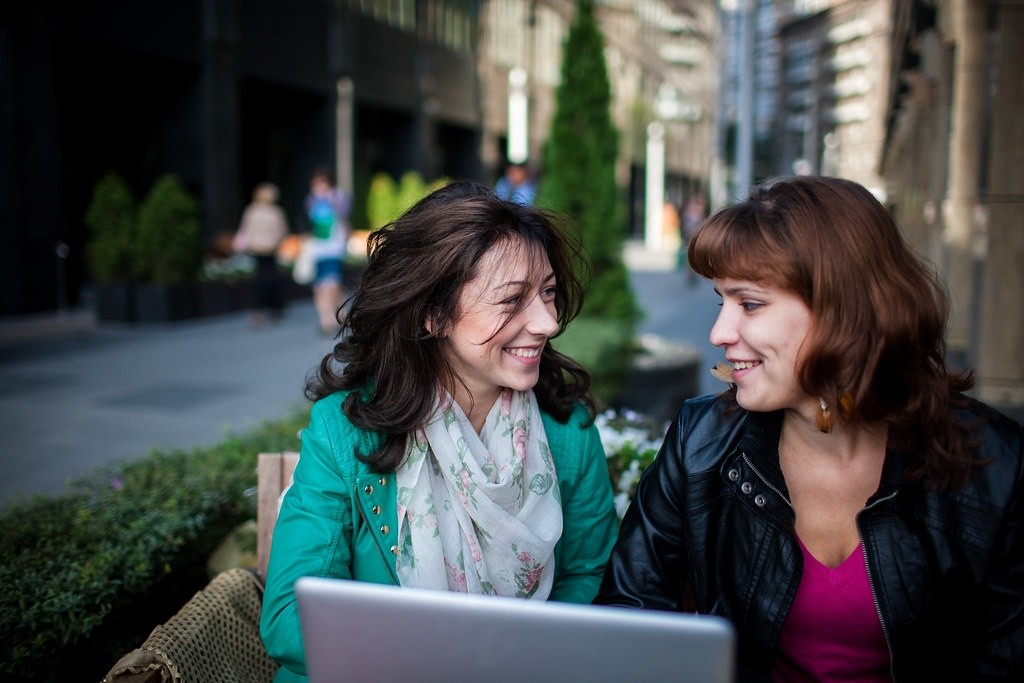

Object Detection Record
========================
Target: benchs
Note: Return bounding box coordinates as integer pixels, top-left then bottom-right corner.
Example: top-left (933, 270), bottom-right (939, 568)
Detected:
top-left (99, 451), bottom-right (300, 680)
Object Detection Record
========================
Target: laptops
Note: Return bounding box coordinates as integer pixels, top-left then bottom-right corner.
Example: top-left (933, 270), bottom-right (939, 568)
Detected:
top-left (296, 577), bottom-right (735, 683)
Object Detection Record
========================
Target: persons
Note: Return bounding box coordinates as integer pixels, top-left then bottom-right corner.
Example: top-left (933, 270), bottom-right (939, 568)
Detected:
top-left (230, 156), bottom-right (540, 334)
top-left (588, 172), bottom-right (1024, 683)
top-left (254, 182), bottom-right (623, 681)
